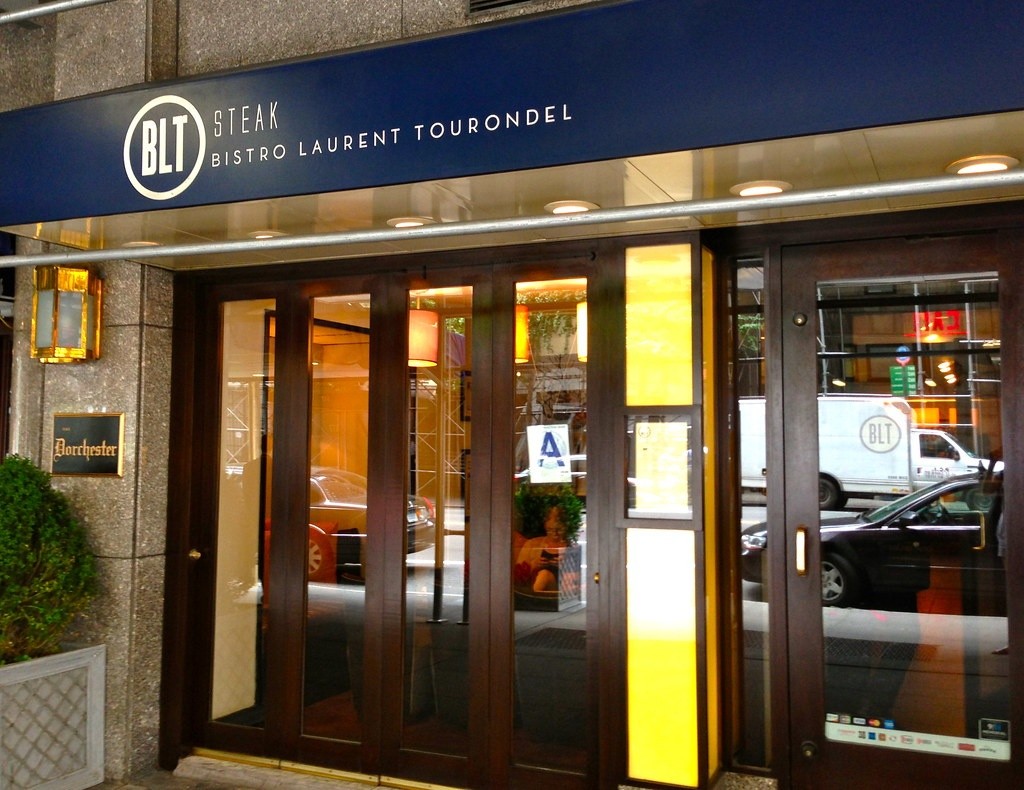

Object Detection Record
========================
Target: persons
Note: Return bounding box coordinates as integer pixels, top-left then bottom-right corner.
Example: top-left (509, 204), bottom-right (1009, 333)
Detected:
top-left (515, 506), bottom-right (581, 599)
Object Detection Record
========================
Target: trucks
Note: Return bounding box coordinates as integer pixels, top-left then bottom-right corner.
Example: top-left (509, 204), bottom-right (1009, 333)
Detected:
top-left (740, 393), bottom-right (1008, 511)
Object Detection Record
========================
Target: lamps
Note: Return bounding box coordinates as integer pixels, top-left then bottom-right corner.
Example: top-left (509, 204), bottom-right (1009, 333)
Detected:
top-left (515, 305), bottom-right (529, 364)
top-left (576, 301), bottom-right (588, 362)
top-left (409, 296), bottom-right (438, 368)
top-left (29, 266), bottom-right (103, 365)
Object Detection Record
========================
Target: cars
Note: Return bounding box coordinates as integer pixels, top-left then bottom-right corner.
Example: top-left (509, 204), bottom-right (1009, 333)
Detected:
top-left (312, 466), bottom-right (436, 568)
top-left (516, 454), bottom-right (636, 504)
top-left (737, 468), bottom-right (1006, 613)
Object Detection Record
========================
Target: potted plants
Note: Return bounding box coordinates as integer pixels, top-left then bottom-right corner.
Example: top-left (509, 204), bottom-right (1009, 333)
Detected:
top-left (0, 452), bottom-right (104, 790)
top-left (514, 478), bottom-right (583, 613)
